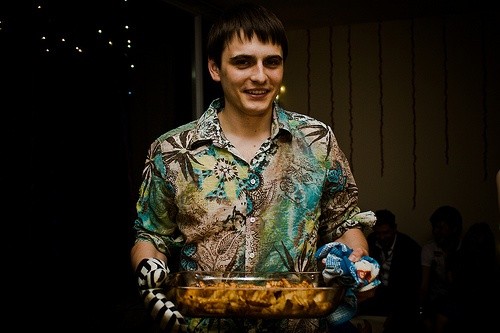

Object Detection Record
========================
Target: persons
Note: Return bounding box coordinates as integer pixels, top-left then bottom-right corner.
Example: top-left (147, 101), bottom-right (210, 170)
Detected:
top-left (130, 3), bottom-right (382, 333)
top-left (328, 205), bottom-right (500, 333)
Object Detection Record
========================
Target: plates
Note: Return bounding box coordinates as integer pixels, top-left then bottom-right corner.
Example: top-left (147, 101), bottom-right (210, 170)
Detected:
top-left (155, 271), bottom-right (346, 318)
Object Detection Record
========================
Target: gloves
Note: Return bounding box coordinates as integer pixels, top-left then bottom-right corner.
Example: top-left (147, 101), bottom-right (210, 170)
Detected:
top-left (134, 257), bottom-right (185, 333)
